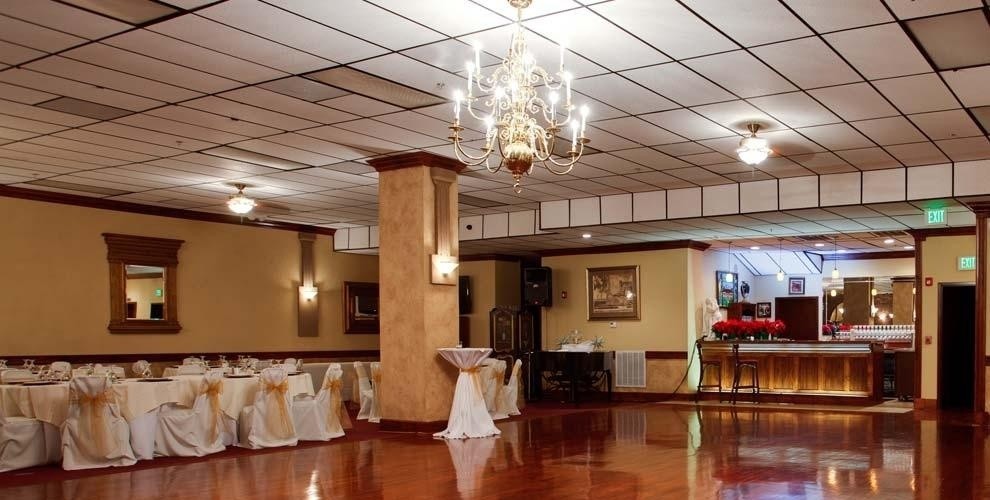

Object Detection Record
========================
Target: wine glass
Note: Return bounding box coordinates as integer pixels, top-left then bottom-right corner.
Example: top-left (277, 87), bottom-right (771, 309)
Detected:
top-left (189, 355), bottom-right (283, 372)
top-left (0, 358), bottom-right (153, 381)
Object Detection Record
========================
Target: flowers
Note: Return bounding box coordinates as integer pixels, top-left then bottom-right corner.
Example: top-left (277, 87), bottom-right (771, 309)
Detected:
top-left (712, 320), bottom-right (785, 340)
top-left (823, 324), bottom-right (836, 335)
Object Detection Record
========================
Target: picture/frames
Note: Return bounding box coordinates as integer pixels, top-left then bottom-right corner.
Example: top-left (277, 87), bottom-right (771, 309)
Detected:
top-left (716, 270), bottom-right (739, 309)
top-left (757, 302), bottom-right (771, 318)
top-left (343, 280), bottom-right (380, 334)
top-left (585, 266), bottom-right (641, 321)
top-left (789, 278), bottom-right (805, 295)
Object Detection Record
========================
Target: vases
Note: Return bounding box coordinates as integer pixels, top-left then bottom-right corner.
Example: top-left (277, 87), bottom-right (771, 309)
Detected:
top-left (741, 280), bottom-right (750, 303)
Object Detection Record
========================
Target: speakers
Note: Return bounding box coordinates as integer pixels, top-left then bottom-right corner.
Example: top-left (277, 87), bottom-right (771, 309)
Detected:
top-left (525, 267), bottom-right (552, 306)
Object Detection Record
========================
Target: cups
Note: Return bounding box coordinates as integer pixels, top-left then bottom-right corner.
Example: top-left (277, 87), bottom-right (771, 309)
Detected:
top-left (455, 341), bottom-right (464, 348)
top-left (834, 325), bottom-right (913, 341)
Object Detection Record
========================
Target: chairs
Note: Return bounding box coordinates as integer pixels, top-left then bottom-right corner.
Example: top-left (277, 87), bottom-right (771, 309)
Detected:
top-left (731, 343), bottom-right (761, 405)
top-left (0, 358), bottom-right (315, 472)
top-left (292, 363), bottom-right (346, 441)
top-left (695, 405), bottom-right (761, 462)
top-left (480, 358), bottom-right (522, 420)
top-left (695, 340), bottom-right (723, 400)
top-left (353, 361), bottom-right (380, 423)
top-left (541, 370), bottom-right (608, 393)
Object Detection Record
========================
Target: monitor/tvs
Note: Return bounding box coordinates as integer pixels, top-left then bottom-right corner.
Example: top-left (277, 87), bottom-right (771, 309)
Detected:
top-left (460, 276), bottom-right (472, 314)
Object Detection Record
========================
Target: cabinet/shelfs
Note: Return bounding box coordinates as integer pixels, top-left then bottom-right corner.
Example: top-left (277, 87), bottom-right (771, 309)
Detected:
top-left (726, 302), bottom-right (756, 321)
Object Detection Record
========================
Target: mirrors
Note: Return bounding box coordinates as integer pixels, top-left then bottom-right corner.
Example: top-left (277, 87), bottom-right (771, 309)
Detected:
top-left (823, 278), bottom-right (844, 335)
top-left (101, 232), bottom-right (185, 335)
top-left (869, 277), bottom-right (893, 326)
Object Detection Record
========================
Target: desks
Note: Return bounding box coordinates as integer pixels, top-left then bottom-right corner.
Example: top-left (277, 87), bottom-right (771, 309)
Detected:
top-left (438, 345), bottom-right (500, 439)
top-left (533, 350), bottom-right (613, 398)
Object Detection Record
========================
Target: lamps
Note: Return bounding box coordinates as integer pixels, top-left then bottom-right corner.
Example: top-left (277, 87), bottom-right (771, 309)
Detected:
top-left (735, 124), bottom-right (773, 165)
top-left (433, 260), bottom-right (457, 278)
top-left (446, 0), bottom-right (593, 194)
top-left (226, 184), bottom-right (257, 215)
top-left (727, 242), bottom-right (734, 282)
top-left (777, 237), bottom-right (785, 282)
top-left (301, 288), bottom-right (316, 303)
top-left (832, 236), bottom-right (838, 279)
